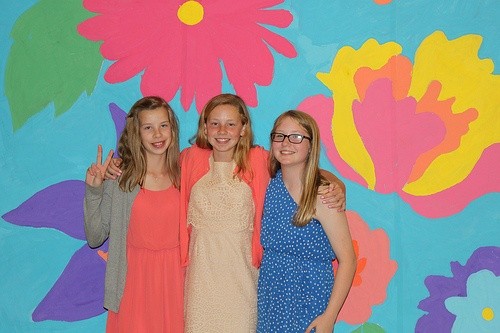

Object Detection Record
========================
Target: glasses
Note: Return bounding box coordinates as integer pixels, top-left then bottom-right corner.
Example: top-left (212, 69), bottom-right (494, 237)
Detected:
top-left (271, 133), bottom-right (311, 144)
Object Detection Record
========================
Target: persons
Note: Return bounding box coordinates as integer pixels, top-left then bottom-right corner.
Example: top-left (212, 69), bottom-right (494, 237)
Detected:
top-left (84, 95), bottom-right (183, 333)
top-left (255, 109), bottom-right (356, 333)
top-left (105, 94), bottom-right (346, 333)
